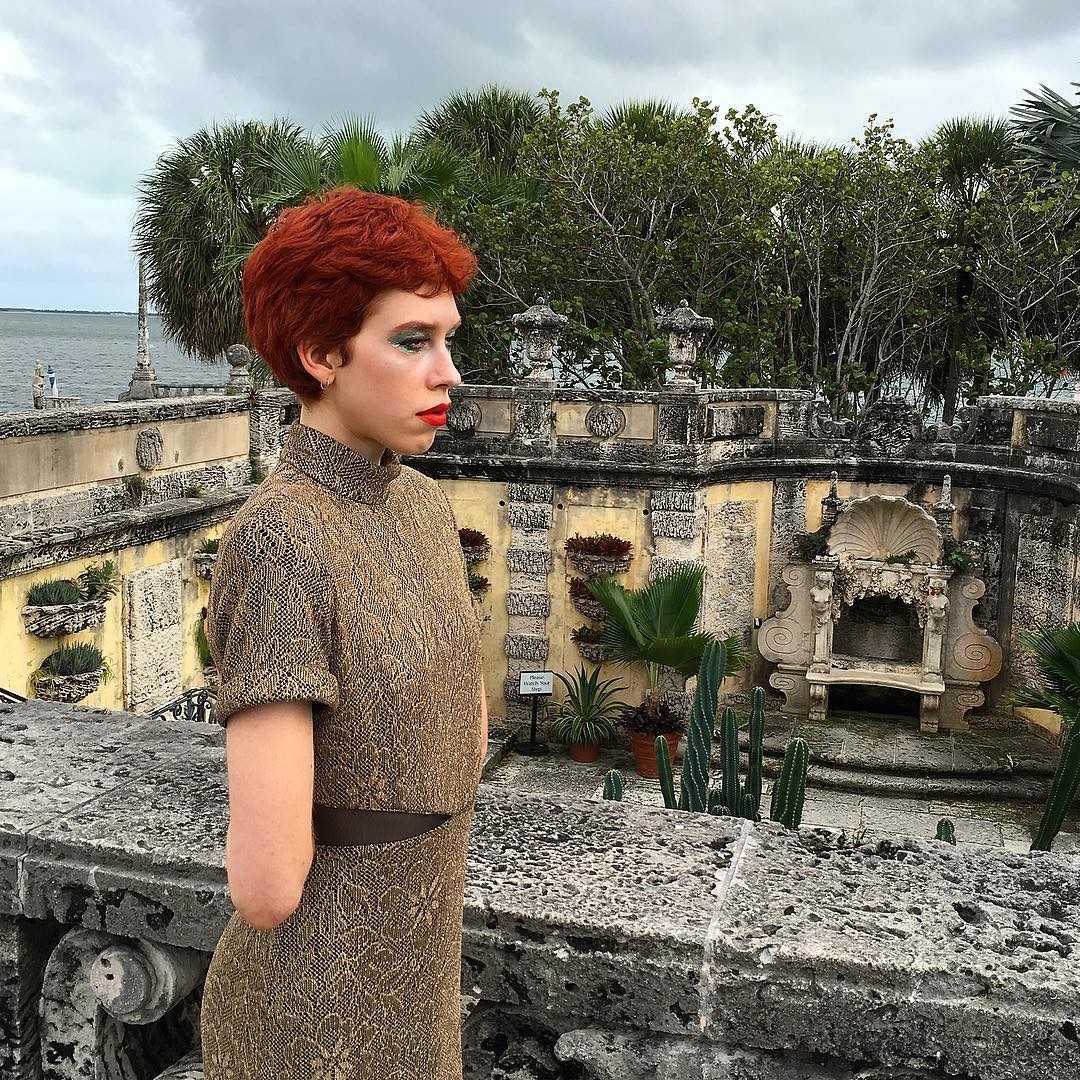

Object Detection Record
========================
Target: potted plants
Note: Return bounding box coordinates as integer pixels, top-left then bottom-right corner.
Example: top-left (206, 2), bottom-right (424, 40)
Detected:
top-left (584, 564), bottom-right (764, 778)
top-left (564, 531), bottom-right (635, 576)
top-left (541, 662), bottom-right (632, 762)
top-left (22, 559), bottom-right (121, 638)
top-left (467, 564), bottom-right (489, 602)
top-left (458, 528), bottom-right (491, 565)
top-left (567, 577), bottom-right (631, 621)
top-left (570, 625), bottom-right (606, 661)
top-left (193, 538), bottom-right (221, 580)
top-left (27, 638), bottom-right (115, 704)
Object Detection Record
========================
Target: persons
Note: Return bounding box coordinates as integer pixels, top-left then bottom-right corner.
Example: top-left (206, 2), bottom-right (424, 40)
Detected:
top-left (199, 185), bottom-right (488, 1078)
